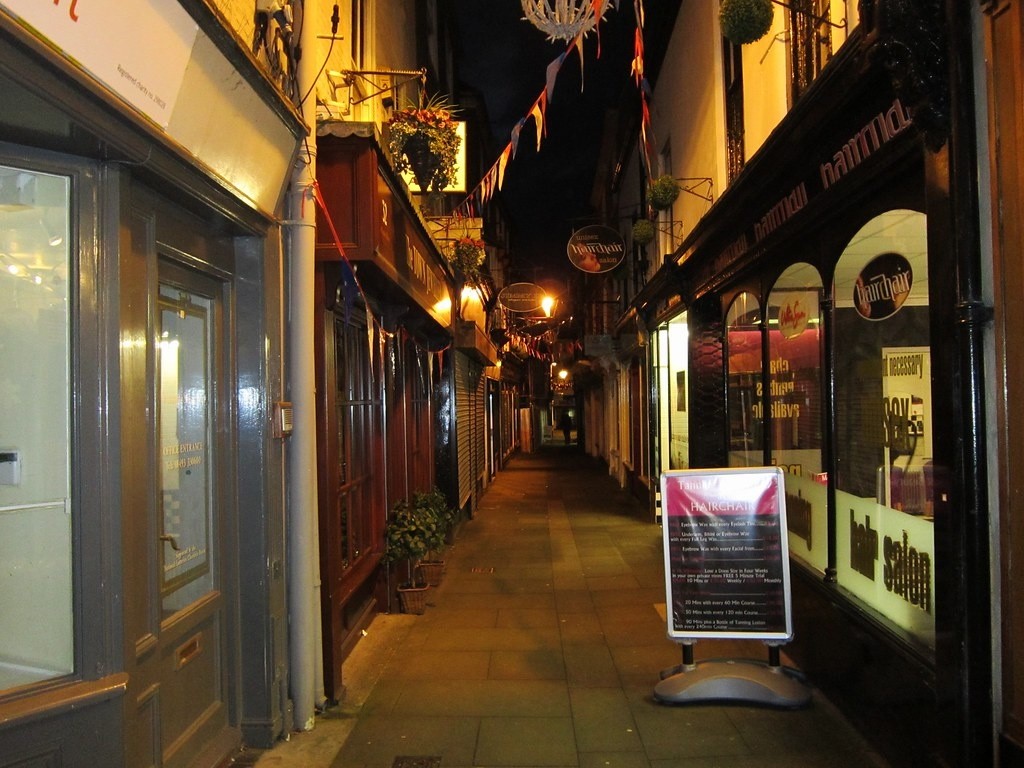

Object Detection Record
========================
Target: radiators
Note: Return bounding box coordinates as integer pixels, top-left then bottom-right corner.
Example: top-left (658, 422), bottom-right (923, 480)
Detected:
top-left (901, 471), bottom-right (921, 514)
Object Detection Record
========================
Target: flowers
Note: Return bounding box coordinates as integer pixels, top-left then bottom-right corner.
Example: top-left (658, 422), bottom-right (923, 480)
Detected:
top-left (447, 231), bottom-right (487, 290)
top-left (387, 85), bottom-right (465, 217)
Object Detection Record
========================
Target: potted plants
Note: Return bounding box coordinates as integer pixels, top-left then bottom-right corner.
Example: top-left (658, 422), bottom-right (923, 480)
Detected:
top-left (384, 488), bottom-right (452, 614)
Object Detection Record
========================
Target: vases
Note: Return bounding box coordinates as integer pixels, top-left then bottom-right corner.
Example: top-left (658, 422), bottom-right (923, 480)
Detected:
top-left (404, 133), bottom-right (438, 194)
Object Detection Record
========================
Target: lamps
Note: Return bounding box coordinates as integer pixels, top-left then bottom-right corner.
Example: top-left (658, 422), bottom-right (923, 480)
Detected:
top-left (38, 206), bottom-right (62, 246)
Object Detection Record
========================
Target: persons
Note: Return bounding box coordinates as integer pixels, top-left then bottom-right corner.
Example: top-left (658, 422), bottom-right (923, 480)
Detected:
top-left (561, 413), bottom-right (571, 442)
